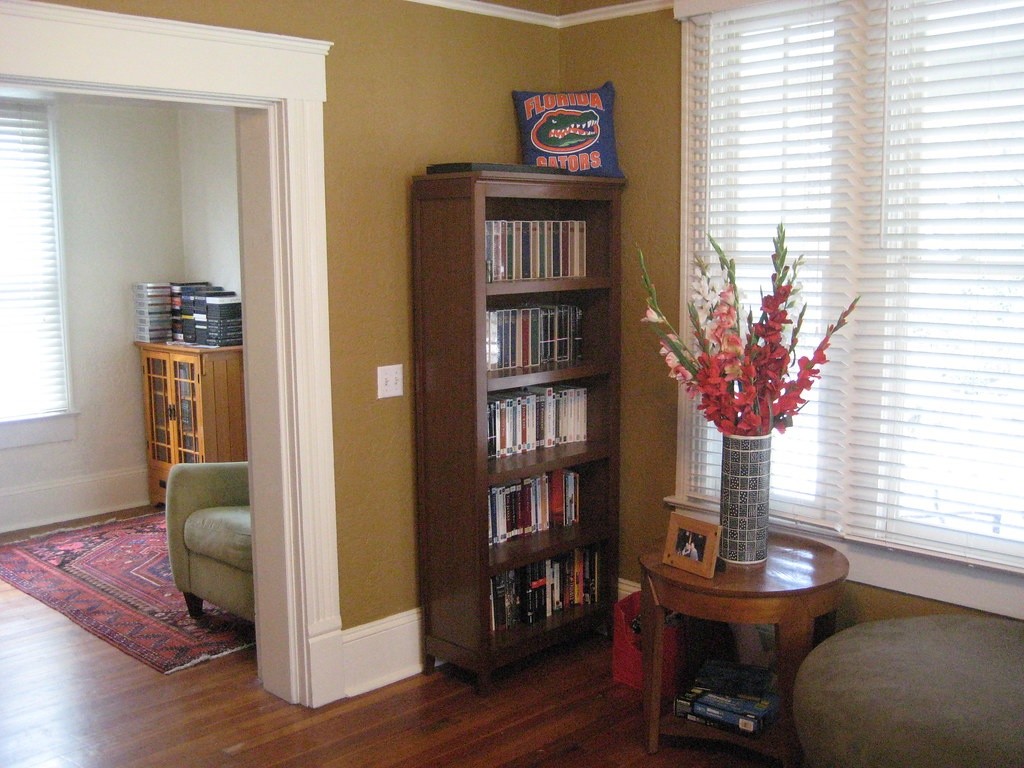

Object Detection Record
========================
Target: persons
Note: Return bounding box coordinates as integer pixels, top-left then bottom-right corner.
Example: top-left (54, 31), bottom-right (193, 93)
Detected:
top-left (676, 542), bottom-right (700, 562)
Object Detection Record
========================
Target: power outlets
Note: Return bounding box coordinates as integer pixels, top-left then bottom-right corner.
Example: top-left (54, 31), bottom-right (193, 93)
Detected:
top-left (376, 364), bottom-right (403, 399)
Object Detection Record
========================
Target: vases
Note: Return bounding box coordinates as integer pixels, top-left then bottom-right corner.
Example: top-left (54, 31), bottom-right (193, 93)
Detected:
top-left (718, 433), bottom-right (772, 566)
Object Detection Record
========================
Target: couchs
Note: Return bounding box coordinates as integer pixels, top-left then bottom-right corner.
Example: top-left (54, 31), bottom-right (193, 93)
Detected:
top-left (165, 461), bottom-right (255, 624)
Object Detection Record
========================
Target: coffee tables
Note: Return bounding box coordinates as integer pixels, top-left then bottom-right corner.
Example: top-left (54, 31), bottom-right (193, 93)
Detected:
top-left (638, 533), bottom-right (849, 767)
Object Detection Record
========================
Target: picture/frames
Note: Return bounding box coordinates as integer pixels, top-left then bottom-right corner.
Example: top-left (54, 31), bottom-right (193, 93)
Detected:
top-left (661, 511), bottom-right (724, 579)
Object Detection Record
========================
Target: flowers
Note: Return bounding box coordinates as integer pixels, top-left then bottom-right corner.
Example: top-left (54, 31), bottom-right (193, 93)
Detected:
top-left (635, 222), bottom-right (861, 438)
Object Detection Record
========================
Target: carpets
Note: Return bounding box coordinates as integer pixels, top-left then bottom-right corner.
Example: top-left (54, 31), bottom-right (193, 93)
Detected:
top-left (0, 508), bottom-right (255, 675)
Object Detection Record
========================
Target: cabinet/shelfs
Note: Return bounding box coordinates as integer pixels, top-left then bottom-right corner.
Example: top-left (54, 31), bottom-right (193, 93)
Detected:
top-left (410, 170), bottom-right (619, 695)
top-left (133, 341), bottom-right (248, 507)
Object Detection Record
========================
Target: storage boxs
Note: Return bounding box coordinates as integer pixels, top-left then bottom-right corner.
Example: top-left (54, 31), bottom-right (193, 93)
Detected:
top-left (613, 589), bottom-right (731, 699)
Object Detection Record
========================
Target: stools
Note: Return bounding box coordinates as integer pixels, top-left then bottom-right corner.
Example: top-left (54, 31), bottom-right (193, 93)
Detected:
top-left (792, 612), bottom-right (1024, 768)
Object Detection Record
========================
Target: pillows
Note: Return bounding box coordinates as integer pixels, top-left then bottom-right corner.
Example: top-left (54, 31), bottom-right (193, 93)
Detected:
top-left (510, 79), bottom-right (625, 179)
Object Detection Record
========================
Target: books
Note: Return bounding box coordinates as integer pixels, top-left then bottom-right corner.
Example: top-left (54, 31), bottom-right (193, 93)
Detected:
top-left (479, 210), bottom-right (597, 635)
